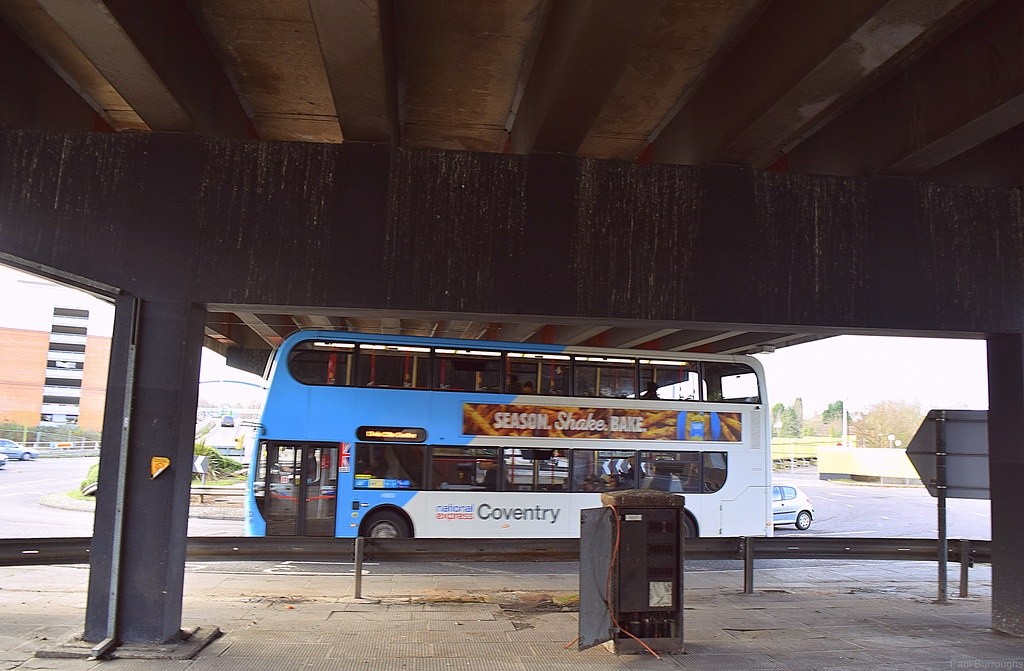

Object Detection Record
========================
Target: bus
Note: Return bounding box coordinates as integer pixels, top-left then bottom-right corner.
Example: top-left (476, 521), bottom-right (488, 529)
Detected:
top-left (244, 327), bottom-right (774, 539)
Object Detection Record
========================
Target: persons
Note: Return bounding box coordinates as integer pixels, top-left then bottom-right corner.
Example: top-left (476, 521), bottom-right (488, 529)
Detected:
top-left (562, 473), bottom-right (619, 493)
top-left (620, 455), bottom-right (644, 480)
top-left (523, 381), bottom-right (533, 393)
top-left (641, 382), bottom-right (660, 399)
top-left (282, 449), bottom-right (317, 481)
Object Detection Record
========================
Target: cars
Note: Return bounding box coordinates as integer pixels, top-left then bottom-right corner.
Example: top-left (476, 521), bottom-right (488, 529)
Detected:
top-left (0, 453), bottom-right (9, 467)
top-left (773, 484), bottom-right (816, 531)
top-left (0, 438), bottom-right (41, 461)
top-left (221, 415), bottom-right (235, 427)
top-left (251, 460), bottom-right (338, 498)
